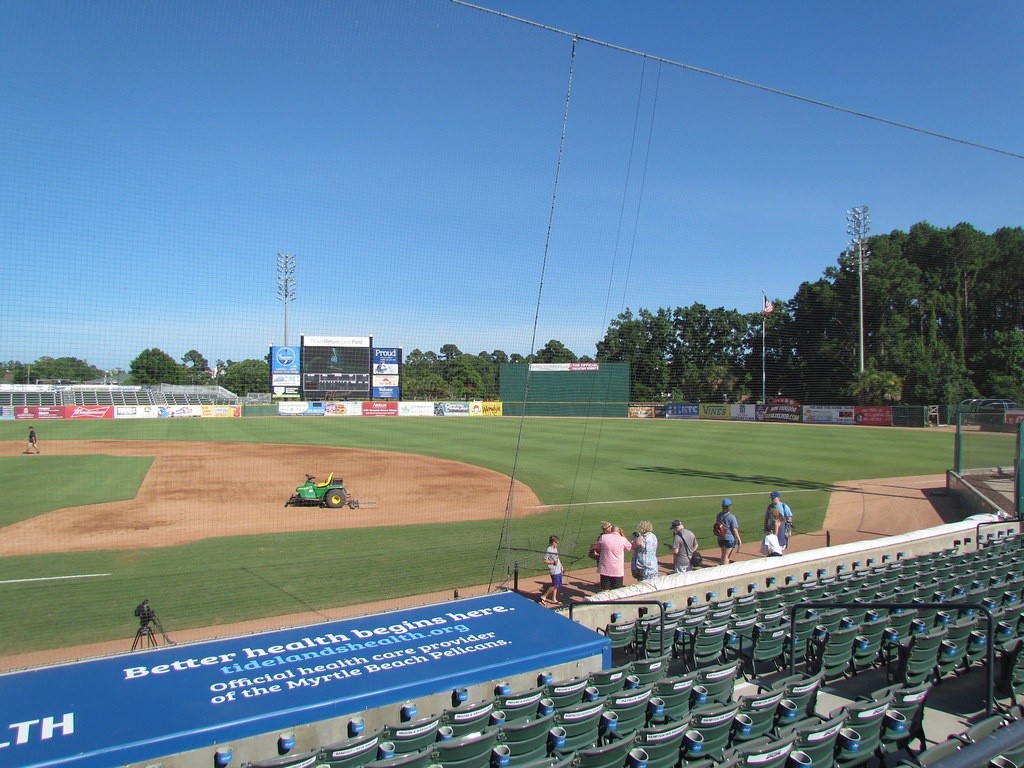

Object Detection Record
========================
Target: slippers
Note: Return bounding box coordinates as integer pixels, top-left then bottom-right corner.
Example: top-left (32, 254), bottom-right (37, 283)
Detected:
top-left (551, 601), bottom-right (562, 605)
top-left (539, 595), bottom-right (546, 605)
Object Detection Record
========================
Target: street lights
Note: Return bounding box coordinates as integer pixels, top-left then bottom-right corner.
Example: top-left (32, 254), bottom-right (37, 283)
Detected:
top-left (276, 249), bottom-right (298, 347)
top-left (845, 204), bottom-right (871, 373)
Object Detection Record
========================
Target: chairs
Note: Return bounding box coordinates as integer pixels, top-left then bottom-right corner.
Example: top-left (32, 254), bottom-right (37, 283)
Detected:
top-left (318, 472), bottom-right (333, 487)
top-left (223, 533), bottom-right (1024, 768)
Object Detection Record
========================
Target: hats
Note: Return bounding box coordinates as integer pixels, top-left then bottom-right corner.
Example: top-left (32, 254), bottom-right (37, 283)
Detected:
top-left (770, 491), bottom-right (779, 499)
top-left (669, 520), bottom-right (682, 530)
top-left (722, 498), bottom-right (732, 506)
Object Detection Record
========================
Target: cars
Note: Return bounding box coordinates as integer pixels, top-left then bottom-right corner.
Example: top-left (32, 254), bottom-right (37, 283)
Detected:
top-left (950, 398), bottom-right (1023, 426)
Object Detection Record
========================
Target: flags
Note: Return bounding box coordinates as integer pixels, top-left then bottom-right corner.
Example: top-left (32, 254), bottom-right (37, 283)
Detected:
top-left (762, 295), bottom-right (775, 314)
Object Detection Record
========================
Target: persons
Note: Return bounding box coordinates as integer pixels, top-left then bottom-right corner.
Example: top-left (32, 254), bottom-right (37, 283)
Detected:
top-left (27, 426), bottom-right (40, 453)
top-left (716, 498), bottom-right (742, 565)
top-left (588, 521), bottom-right (633, 591)
top-left (630, 519), bottom-right (658, 581)
top-left (762, 491), bottom-right (795, 557)
top-left (541, 535), bottom-right (564, 605)
top-left (667, 520), bottom-right (698, 574)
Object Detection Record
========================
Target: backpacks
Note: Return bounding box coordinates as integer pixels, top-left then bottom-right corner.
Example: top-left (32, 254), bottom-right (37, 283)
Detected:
top-left (713, 513), bottom-right (731, 537)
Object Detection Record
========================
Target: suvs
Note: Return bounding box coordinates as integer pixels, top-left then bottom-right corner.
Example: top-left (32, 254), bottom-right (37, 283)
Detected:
top-left (285, 472), bottom-right (351, 509)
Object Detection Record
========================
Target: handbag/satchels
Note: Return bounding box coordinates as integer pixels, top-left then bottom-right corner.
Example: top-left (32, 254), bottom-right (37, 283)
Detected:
top-left (690, 551), bottom-right (702, 567)
top-left (632, 568), bottom-right (647, 581)
top-left (782, 504), bottom-right (792, 538)
top-left (589, 549), bottom-right (600, 561)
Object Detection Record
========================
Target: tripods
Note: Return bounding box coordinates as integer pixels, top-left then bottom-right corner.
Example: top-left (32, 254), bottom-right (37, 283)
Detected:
top-left (132, 626), bottom-right (158, 651)
